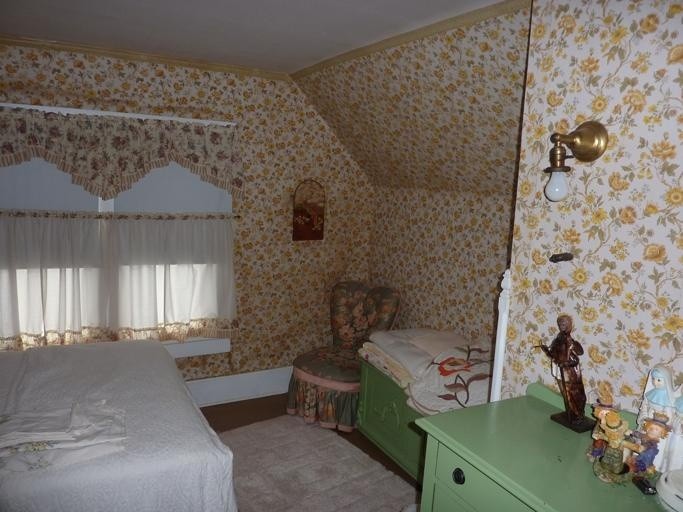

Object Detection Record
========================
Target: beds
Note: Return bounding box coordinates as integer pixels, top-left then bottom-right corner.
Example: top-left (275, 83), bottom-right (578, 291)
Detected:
top-left (0, 337), bottom-right (236, 512)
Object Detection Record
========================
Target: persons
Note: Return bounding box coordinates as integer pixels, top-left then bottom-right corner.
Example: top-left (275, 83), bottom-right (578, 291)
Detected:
top-left (585, 363), bottom-right (683, 496)
top-left (537, 315), bottom-right (588, 423)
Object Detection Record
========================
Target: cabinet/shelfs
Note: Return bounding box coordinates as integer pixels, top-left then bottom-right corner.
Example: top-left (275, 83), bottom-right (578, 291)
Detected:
top-left (414, 385), bottom-right (537, 512)
top-left (355, 356), bottom-right (427, 488)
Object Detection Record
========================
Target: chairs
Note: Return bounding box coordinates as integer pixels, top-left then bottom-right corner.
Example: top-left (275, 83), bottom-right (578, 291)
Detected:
top-left (291, 280), bottom-right (402, 439)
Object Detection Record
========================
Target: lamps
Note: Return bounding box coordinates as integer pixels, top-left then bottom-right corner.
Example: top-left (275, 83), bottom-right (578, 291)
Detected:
top-left (543, 121), bottom-right (609, 202)
